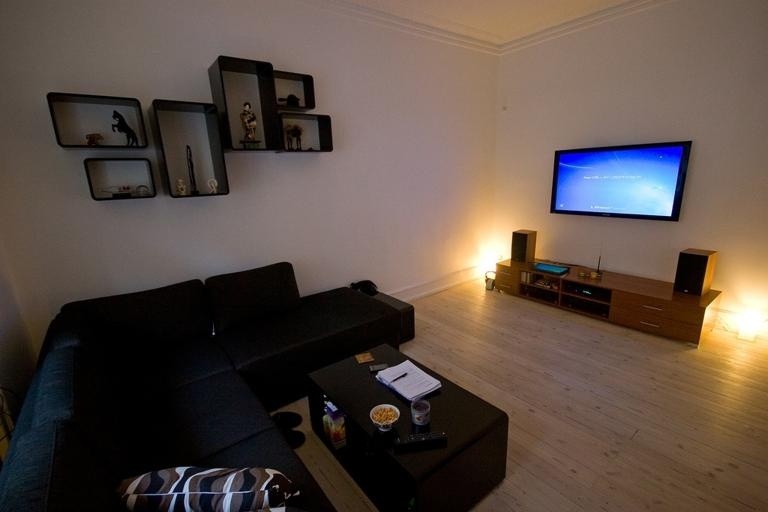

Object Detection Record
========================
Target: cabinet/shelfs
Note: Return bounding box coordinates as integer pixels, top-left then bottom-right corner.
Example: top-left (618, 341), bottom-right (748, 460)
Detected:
top-left (273, 70), bottom-right (315, 112)
top-left (46, 92), bottom-right (148, 148)
top-left (208, 57), bottom-right (283, 151)
top-left (495, 258), bottom-right (723, 349)
top-left (276, 112), bottom-right (331, 153)
top-left (84, 158), bottom-right (157, 198)
top-left (149, 98), bottom-right (230, 198)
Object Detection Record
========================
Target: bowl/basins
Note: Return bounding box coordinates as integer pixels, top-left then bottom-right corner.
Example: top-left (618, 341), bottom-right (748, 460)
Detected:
top-left (368, 403), bottom-right (400, 432)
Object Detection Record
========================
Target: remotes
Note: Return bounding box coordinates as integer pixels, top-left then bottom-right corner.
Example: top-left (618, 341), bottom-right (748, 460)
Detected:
top-left (401, 429), bottom-right (450, 444)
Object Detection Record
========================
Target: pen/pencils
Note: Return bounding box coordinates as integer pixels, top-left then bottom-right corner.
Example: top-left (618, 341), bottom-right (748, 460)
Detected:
top-left (390, 373), bottom-right (407, 383)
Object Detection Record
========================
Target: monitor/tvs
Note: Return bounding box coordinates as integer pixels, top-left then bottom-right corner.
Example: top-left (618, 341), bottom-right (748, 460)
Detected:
top-left (550, 140), bottom-right (693, 221)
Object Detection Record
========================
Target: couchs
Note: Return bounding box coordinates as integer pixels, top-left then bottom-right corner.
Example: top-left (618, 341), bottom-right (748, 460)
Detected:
top-left (0, 262), bottom-right (298, 512)
top-left (0, 262), bottom-right (415, 512)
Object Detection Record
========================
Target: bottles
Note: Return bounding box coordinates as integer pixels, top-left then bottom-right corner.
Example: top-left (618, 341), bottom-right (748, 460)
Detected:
top-left (94, 184), bottom-right (135, 194)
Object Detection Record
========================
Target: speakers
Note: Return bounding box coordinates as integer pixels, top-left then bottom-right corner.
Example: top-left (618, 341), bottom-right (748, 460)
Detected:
top-left (510, 229), bottom-right (537, 265)
top-left (672, 247), bottom-right (717, 297)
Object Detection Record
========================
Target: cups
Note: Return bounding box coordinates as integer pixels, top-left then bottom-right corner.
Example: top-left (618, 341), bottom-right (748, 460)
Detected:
top-left (410, 398), bottom-right (431, 426)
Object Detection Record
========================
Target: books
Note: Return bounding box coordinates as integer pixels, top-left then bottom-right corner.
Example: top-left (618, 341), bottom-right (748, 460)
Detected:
top-left (376, 359), bottom-right (443, 403)
